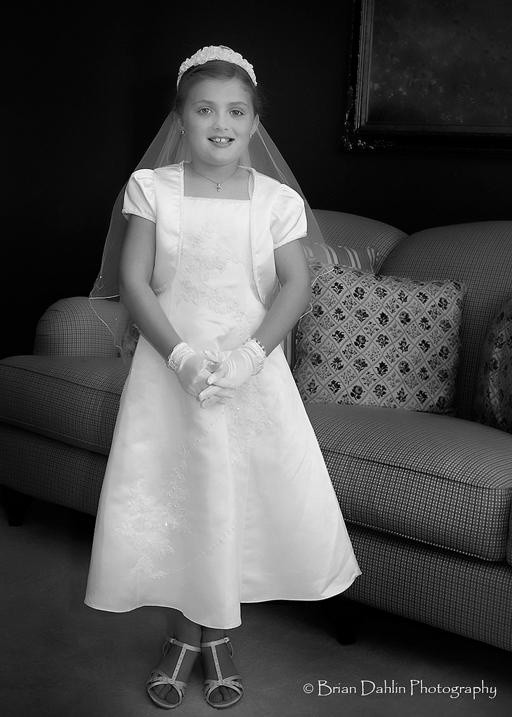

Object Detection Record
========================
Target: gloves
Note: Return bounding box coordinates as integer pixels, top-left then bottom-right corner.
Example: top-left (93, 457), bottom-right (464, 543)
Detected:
top-left (166, 338), bottom-right (265, 409)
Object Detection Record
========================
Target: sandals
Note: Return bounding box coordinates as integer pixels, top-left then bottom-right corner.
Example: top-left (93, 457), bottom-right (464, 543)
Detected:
top-left (146, 635), bottom-right (202, 709)
top-left (198, 638), bottom-right (243, 708)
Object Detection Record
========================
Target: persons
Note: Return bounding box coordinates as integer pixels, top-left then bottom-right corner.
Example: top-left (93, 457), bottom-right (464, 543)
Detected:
top-left (86, 44), bottom-right (362, 710)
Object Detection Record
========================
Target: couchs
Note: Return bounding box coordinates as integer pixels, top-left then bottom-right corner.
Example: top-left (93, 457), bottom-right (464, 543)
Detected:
top-left (1, 207), bottom-right (512, 652)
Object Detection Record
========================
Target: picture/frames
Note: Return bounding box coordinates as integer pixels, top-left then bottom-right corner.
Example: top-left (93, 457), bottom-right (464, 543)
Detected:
top-left (340, 0), bottom-right (512, 153)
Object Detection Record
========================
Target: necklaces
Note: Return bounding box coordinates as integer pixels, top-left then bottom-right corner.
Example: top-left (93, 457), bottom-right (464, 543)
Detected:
top-left (190, 167), bottom-right (238, 192)
